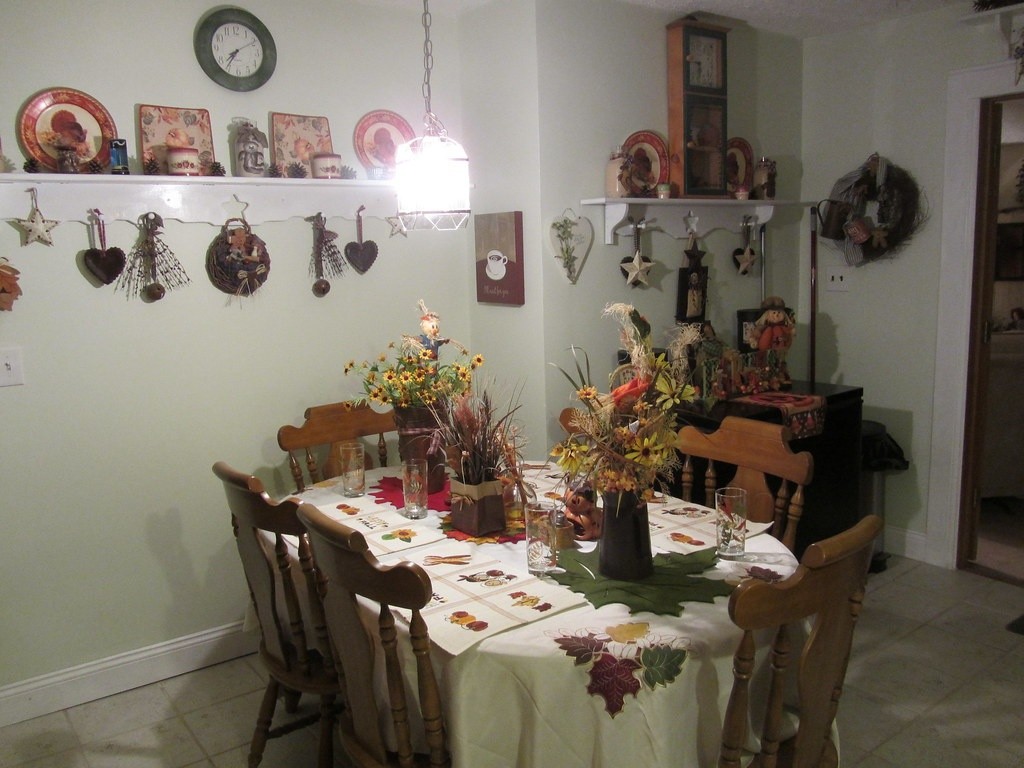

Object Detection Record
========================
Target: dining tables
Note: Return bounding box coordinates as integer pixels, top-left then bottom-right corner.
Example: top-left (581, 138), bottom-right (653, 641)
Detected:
top-left (261, 449), bottom-right (840, 767)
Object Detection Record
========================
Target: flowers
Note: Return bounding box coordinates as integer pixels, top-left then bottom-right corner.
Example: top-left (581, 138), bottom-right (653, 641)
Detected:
top-left (545, 304), bottom-right (701, 518)
top-left (340, 300), bottom-right (484, 408)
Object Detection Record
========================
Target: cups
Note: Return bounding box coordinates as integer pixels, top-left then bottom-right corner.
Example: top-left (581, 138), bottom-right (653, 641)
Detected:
top-left (523, 501), bottom-right (557, 572)
top-left (312, 153), bottom-right (341, 178)
top-left (167, 148), bottom-right (200, 176)
top-left (401, 459), bottom-right (428, 519)
top-left (339, 443), bottom-right (365, 497)
top-left (714, 487), bottom-right (746, 556)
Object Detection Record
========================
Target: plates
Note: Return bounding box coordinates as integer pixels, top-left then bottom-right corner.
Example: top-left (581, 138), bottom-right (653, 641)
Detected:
top-left (727, 137), bottom-right (753, 195)
top-left (622, 130), bottom-right (670, 197)
top-left (354, 110), bottom-right (417, 167)
top-left (139, 104), bottom-right (215, 175)
top-left (19, 88), bottom-right (118, 174)
top-left (272, 112), bottom-right (333, 179)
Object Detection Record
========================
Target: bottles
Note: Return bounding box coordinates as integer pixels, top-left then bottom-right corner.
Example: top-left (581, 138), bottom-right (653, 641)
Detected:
top-left (754, 157), bottom-right (776, 201)
top-left (605, 145), bottom-right (633, 198)
top-left (56, 145), bottom-right (79, 173)
top-left (734, 186), bottom-right (750, 200)
top-left (235, 119), bottom-right (266, 178)
top-left (655, 183), bottom-right (671, 199)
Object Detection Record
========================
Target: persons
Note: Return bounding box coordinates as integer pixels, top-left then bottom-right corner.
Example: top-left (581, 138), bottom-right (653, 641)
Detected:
top-left (411, 313), bottom-right (450, 380)
top-left (750, 297), bottom-right (797, 386)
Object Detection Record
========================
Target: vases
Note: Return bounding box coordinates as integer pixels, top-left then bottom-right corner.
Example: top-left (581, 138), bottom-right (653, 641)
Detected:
top-left (600, 487), bottom-right (654, 582)
top-left (398, 403), bottom-right (449, 493)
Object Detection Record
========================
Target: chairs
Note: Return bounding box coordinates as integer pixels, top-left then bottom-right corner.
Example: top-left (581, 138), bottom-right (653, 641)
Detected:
top-left (213, 393), bottom-right (884, 768)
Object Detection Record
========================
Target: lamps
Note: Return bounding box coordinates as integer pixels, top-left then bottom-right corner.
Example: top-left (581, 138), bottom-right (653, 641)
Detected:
top-left (394, 0), bottom-right (470, 230)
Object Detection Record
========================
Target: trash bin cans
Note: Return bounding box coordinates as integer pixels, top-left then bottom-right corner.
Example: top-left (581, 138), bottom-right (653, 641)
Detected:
top-left (862, 419), bottom-right (891, 573)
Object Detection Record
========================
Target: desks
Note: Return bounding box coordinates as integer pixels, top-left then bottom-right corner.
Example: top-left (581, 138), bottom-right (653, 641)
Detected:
top-left (667, 379), bottom-right (866, 554)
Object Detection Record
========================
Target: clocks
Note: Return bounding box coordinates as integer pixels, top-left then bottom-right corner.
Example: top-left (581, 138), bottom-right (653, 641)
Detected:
top-left (664, 18), bottom-right (731, 199)
top-left (195, 8), bottom-right (277, 93)
